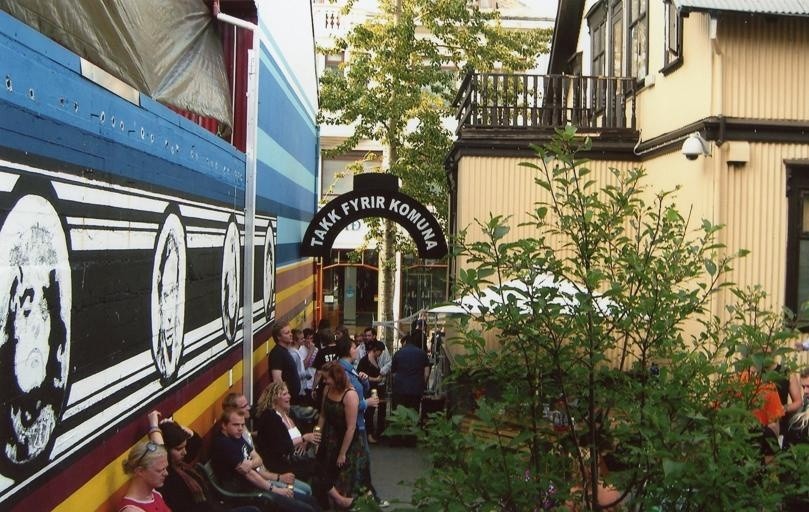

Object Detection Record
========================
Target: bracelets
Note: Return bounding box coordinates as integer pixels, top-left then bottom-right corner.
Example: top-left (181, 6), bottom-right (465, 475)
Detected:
top-left (277, 474), bottom-right (280, 481)
top-left (302, 437), bottom-right (306, 443)
top-left (148, 426), bottom-right (157, 431)
top-left (784, 404), bottom-right (788, 413)
top-left (268, 483), bottom-right (273, 491)
top-left (148, 429), bottom-right (162, 440)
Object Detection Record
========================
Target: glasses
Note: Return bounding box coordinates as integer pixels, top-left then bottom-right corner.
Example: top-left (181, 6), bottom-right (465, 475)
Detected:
top-left (140, 441), bottom-right (158, 460)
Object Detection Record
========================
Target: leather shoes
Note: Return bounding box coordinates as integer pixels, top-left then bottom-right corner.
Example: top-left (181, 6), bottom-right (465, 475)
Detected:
top-left (335, 497), bottom-right (356, 510)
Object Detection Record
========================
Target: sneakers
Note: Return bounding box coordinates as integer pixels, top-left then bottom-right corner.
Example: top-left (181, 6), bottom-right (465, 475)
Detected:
top-left (373, 497), bottom-right (390, 508)
top-left (368, 435), bottom-right (377, 444)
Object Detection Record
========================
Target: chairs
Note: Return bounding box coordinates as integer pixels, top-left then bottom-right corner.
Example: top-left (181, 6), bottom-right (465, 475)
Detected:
top-left (197, 459), bottom-right (272, 501)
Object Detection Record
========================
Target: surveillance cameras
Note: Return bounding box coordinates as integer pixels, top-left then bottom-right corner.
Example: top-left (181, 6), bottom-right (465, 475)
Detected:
top-left (681, 133), bottom-right (703, 160)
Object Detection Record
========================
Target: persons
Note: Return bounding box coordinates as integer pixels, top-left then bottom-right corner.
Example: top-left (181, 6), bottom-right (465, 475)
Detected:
top-left (759, 342), bottom-right (803, 435)
top-left (390, 314), bottom-right (430, 448)
top-left (708, 344), bottom-right (784, 436)
top-left (287, 319), bottom-right (364, 406)
top-left (744, 422), bottom-right (780, 468)
top-left (0, 226), bottom-right (69, 464)
top-left (224, 236), bottom-right (239, 337)
top-left (264, 241), bottom-right (276, 317)
top-left (268, 320), bottom-right (301, 406)
top-left (317, 361), bottom-right (364, 498)
top-left (222, 392), bottom-right (311, 496)
top-left (212, 407), bottom-right (321, 511)
top-left (357, 328), bottom-right (393, 437)
top-left (782, 366), bottom-right (809, 455)
top-left (117, 439), bottom-right (170, 512)
top-left (357, 339), bottom-right (385, 444)
top-left (152, 231), bottom-right (183, 377)
top-left (148, 410), bottom-right (260, 511)
top-left (336, 338), bottom-right (390, 508)
top-left (258, 381), bottom-right (358, 511)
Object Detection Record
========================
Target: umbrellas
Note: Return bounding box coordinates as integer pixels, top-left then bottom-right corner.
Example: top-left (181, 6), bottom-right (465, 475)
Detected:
top-left (429, 268), bottom-right (649, 396)
